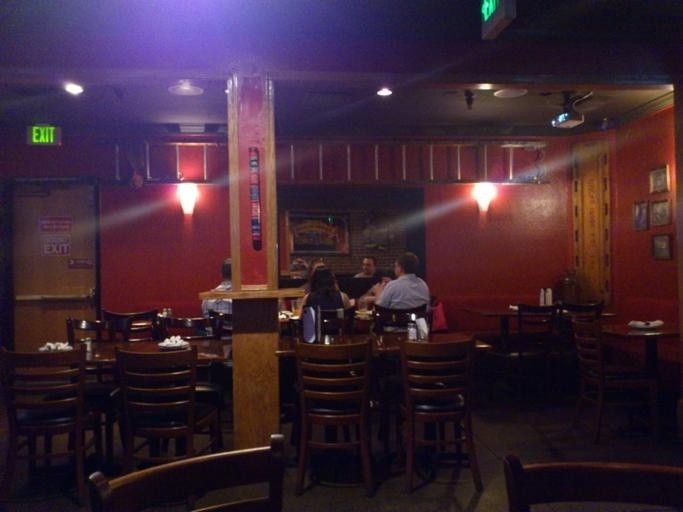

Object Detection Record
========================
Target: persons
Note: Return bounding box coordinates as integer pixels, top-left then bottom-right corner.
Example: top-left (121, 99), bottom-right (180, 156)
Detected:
top-left (353, 255), bottom-right (377, 278)
top-left (373, 251), bottom-right (430, 333)
top-left (299, 262), bottom-right (352, 320)
top-left (201, 258), bottom-right (232, 367)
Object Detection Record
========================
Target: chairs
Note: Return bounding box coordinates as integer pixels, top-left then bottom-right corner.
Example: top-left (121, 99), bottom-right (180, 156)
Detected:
top-left (501, 453), bottom-right (683, 512)
top-left (397, 335), bottom-right (482, 493)
top-left (89, 434), bottom-right (286, 511)
top-left (294, 337), bottom-right (375, 498)
top-left (114, 345), bottom-right (223, 476)
top-left (1, 344), bottom-right (102, 506)
top-left (463, 300), bottom-right (682, 444)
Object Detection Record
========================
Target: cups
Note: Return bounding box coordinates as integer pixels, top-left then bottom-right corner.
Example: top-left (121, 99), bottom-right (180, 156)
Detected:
top-left (417, 324), bottom-right (430, 343)
top-left (292, 299), bottom-right (304, 317)
top-left (357, 294), bottom-right (368, 312)
top-left (367, 296), bottom-right (375, 311)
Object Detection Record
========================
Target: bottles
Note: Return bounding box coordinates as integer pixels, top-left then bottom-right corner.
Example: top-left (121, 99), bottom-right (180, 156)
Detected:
top-left (292, 290), bottom-right (308, 316)
top-left (407, 322), bottom-right (418, 341)
top-left (80, 337), bottom-right (91, 353)
top-left (162, 308), bottom-right (173, 317)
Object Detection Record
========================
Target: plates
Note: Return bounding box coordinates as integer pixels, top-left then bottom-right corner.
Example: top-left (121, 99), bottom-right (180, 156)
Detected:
top-left (158, 342), bottom-right (190, 349)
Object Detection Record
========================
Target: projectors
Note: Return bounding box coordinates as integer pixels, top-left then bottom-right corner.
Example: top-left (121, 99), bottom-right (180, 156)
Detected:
top-left (550, 111), bottom-right (585, 129)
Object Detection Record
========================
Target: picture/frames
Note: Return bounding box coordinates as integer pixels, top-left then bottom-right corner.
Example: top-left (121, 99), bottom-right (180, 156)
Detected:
top-left (631, 164), bottom-right (672, 261)
top-left (286, 212), bottom-right (353, 256)
